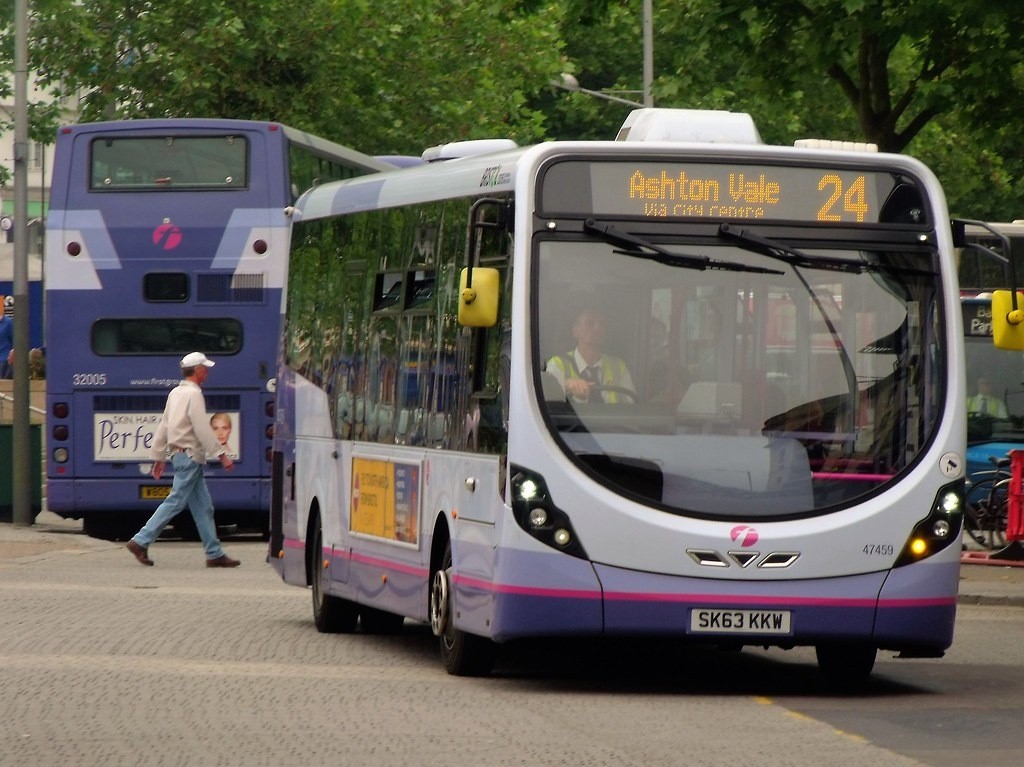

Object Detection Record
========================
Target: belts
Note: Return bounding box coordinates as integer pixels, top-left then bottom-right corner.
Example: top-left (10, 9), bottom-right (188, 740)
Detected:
top-left (179, 448), bottom-right (187, 452)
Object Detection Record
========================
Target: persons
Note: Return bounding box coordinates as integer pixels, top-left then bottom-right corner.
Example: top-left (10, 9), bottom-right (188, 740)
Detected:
top-left (964, 370), bottom-right (1008, 420)
top-left (124, 351), bottom-right (242, 568)
top-left (545, 303), bottom-right (636, 406)
top-left (0, 296), bottom-right (15, 379)
top-left (209, 412), bottom-right (236, 460)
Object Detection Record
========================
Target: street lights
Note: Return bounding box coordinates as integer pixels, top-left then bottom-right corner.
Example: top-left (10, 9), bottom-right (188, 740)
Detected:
top-left (547, 73), bottom-right (654, 114)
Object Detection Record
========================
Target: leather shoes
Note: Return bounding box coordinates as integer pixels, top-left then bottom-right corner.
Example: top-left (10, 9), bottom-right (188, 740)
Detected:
top-left (125, 539), bottom-right (154, 566)
top-left (206, 554), bottom-right (241, 568)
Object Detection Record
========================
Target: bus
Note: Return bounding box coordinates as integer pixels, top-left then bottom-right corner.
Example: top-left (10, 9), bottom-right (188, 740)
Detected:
top-left (637, 216), bottom-right (1024, 511)
top-left (40, 117), bottom-right (442, 541)
top-left (264, 107), bottom-right (1024, 686)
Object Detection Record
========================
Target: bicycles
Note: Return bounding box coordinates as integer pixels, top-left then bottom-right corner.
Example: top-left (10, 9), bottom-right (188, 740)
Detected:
top-left (959, 450), bottom-right (1023, 556)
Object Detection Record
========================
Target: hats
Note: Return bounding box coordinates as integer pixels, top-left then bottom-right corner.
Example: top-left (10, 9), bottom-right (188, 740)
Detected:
top-left (179, 352), bottom-right (216, 368)
top-left (976, 368), bottom-right (993, 382)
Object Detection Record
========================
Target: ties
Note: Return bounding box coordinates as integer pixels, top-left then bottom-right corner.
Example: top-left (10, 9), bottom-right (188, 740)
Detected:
top-left (980, 397), bottom-right (988, 417)
top-left (586, 365), bottom-right (604, 404)
